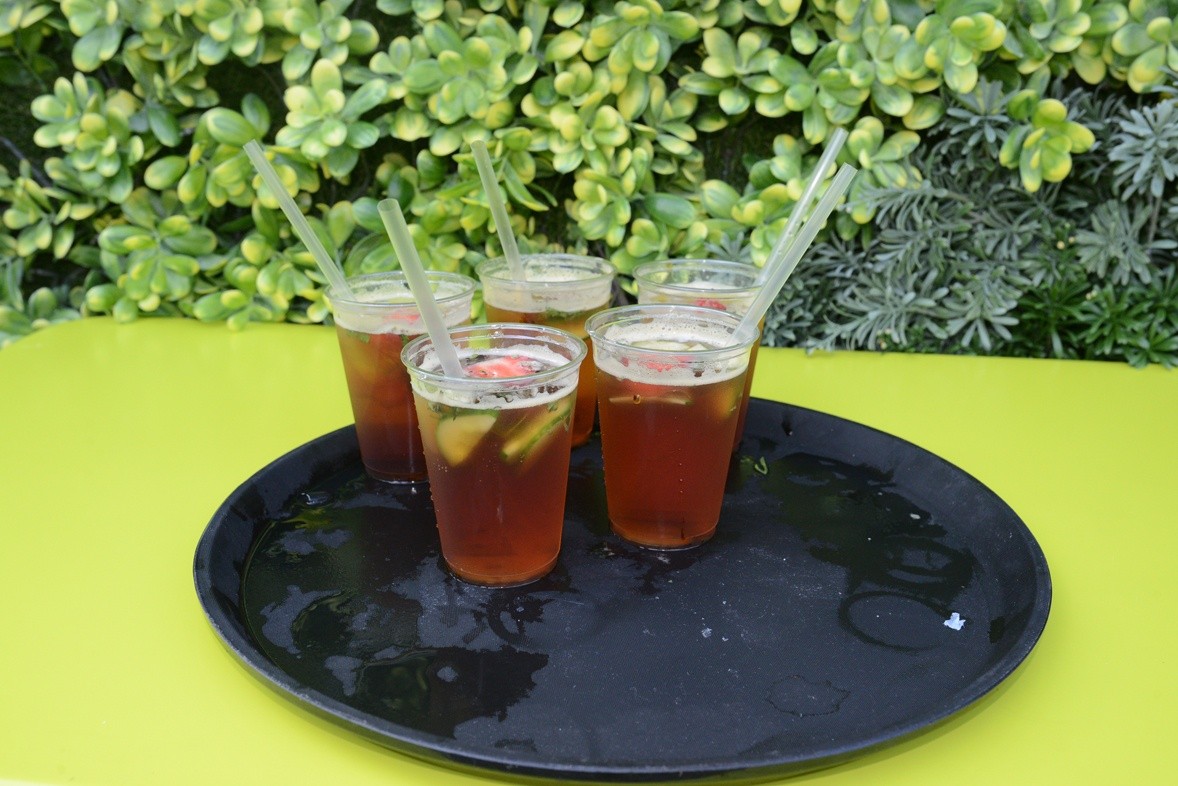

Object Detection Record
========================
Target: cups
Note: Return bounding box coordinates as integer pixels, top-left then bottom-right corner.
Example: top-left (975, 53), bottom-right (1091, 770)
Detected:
top-left (586, 304), bottom-right (759, 552)
top-left (475, 254), bottom-right (617, 449)
top-left (400, 322), bottom-right (586, 586)
top-left (325, 269), bottom-right (478, 485)
top-left (632, 259), bottom-right (770, 452)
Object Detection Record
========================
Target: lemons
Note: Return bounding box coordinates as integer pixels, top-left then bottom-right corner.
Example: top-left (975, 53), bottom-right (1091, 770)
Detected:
top-left (344, 308), bottom-right (741, 476)
